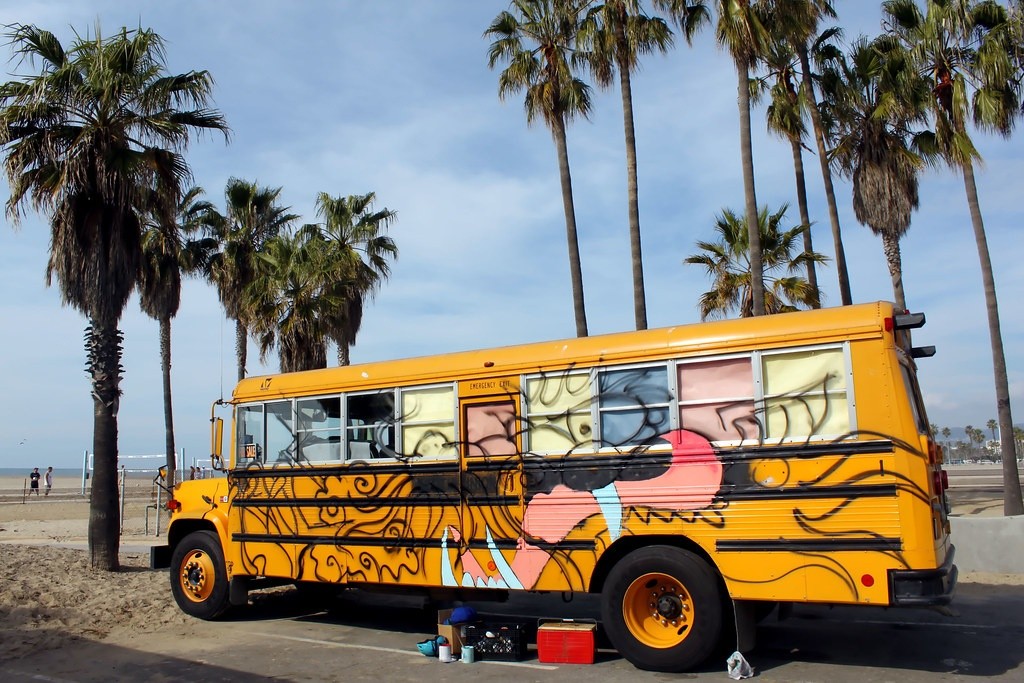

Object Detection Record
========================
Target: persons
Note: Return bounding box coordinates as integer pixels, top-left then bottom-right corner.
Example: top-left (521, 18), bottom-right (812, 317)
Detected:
top-left (118, 465), bottom-right (125, 485)
top-left (190, 466), bottom-right (214, 480)
top-left (29, 468), bottom-right (42, 495)
top-left (43, 467), bottom-right (52, 495)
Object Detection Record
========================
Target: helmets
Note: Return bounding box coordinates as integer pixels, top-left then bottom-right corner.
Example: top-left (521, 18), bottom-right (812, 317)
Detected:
top-left (417, 635), bottom-right (446, 656)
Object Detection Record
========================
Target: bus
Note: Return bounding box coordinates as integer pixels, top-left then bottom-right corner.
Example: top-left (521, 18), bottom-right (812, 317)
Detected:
top-left (151, 300), bottom-right (959, 673)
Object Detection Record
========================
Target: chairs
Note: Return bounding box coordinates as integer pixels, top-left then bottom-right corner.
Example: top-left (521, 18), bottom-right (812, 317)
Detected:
top-left (301, 439), bottom-right (380, 461)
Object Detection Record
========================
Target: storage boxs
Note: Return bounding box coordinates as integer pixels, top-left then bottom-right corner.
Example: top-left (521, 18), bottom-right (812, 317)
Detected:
top-left (438, 609), bottom-right (466, 654)
top-left (536, 616), bottom-right (597, 664)
top-left (466, 622), bottom-right (528, 661)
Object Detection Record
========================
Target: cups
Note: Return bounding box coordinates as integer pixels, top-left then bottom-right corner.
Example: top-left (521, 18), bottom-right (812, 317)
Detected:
top-left (462, 646), bottom-right (474, 664)
top-left (439, 645), bottom-right (451, 663)
